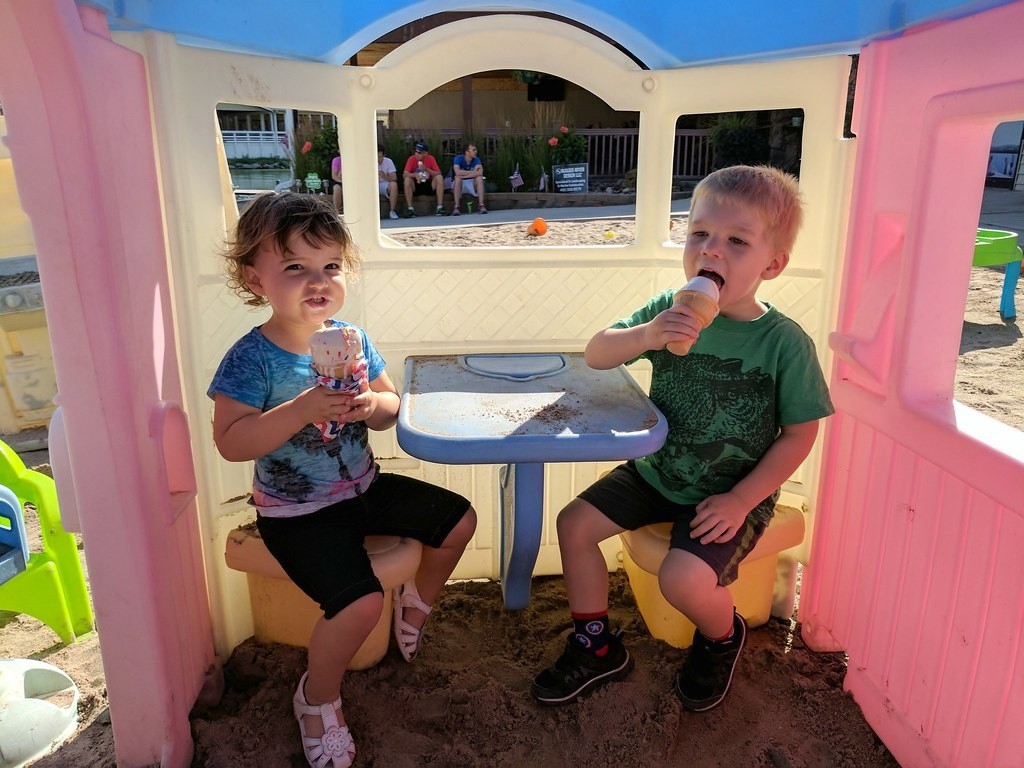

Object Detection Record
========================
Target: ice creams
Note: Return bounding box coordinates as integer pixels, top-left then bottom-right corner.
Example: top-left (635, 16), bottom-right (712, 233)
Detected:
top-left (308, 325), bottom-right (363, 380)
top-left (667, 276), bottom-right (719, 355)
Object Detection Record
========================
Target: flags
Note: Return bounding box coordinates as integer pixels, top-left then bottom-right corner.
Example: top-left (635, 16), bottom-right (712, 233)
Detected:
top-left (509, 169), bottom-right (524, 188)
top-left (538, 168), bottom-right (546, 191)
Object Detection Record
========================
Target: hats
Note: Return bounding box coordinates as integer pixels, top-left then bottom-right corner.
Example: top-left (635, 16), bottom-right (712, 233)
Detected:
top-left (412, 143), bottom-right (429, 152)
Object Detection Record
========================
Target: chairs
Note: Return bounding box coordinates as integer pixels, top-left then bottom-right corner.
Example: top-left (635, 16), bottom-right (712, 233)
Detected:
top-left (0, 441), bottom-right (91, 645)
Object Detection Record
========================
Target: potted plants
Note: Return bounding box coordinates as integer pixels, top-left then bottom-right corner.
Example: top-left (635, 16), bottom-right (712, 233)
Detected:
top-left (707, 113), bottom-right (759, 170)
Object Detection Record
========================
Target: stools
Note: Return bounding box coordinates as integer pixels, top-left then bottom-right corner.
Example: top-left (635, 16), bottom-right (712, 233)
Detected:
top-left (224, 518), bottom-right (420, 671)
top-left (620, 500), bottom-right (805, 646)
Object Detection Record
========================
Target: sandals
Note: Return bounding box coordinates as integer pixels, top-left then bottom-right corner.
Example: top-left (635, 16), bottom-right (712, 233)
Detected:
top-left (292, 671), bottom-right (356, 768)
top-left (393, 579), bottom-right (435, 664)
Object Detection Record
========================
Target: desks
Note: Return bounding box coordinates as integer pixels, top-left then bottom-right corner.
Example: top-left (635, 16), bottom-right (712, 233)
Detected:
top-left (392, 353), bottom-right (668, 613)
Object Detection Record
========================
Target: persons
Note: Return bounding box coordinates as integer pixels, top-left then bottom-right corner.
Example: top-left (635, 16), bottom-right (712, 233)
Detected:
top-left (528, 166), bottom-right (836, 712)
top-left (205, 193), bottom-right (477, 768)
top-left (452, 142), bottom-right (489, 216)
top-left (331, 156), bottom-right (343, 215)
top-left (402, 142), bottom-right (449, 219)
top-left (378, 144), bottom-right (400, 219)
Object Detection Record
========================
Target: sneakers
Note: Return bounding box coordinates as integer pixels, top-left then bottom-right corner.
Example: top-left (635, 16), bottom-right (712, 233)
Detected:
top-left (389, 211), bottom-right (399, 219)
top-left (529, 630), bottom-right (634, 706)
top-left (403, 209), bottom-right (416, 219)
top-left (451, 207), bottom-right (460, 216)
top-left (436, 208), bottom-right (444, 217)
top-left (478, 204), bottom-right (487, 213)
top-left (676, 609), bottom-right (748, 712)
